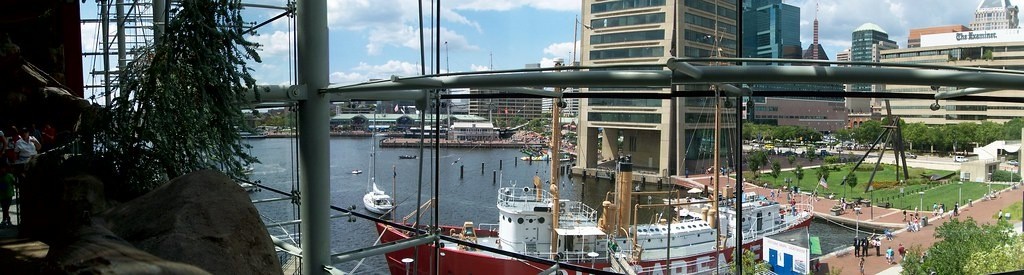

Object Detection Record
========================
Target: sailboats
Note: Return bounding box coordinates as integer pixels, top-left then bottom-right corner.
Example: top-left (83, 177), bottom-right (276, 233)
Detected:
top-left (362, 110), bottom-right (394, 216)
top-left (375, 57), bottom-right (816, 275)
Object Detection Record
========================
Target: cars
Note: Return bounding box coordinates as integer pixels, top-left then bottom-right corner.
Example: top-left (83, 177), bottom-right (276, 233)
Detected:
top-left (1007, 160), bottom-right (1018, 166)
top-left (835, 142), bottom-right (884, 151)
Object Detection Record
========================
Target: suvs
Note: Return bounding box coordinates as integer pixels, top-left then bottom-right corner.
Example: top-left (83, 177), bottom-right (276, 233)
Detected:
top-left (900, 152), bottom-right (917, 159)
top-left (953, 156), bottom-right (969, 162)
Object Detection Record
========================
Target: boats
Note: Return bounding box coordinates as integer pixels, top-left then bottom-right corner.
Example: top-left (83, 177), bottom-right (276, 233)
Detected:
top-left (398, 154), bottom-right (416, 161)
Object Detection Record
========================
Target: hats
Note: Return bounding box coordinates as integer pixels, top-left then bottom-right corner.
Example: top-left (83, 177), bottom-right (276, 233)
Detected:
top-left (21, 127), bottom-right (30, 133)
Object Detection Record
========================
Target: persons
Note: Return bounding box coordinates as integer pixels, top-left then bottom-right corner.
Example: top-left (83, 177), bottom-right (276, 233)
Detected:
top-left (608, 238), bottom-right (621, 256)
top-left (685, 166), bottom-right (1004, 275)
top-left (0, 120), bottom-right (55, 226)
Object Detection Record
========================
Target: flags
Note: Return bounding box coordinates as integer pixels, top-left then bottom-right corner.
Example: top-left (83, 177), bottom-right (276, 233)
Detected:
top-left (820, 178), bottom-right (828, 188)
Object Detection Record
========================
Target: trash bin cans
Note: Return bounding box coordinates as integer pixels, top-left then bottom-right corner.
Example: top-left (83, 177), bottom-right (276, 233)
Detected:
top-left (1020, 180), bottom-right (1023, 186)
top-left (968, 199), bottom-right (972, 207)
top-left (921, 216), bottom-right (928, 226)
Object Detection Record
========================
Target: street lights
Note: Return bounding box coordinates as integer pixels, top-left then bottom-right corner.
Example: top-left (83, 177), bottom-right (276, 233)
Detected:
top-left (957, 182), bottom-right (963, 208)
top-left (842, 175), bottom-right (848, 199)
top-left (988, 172), bottom-right (992, 196)
top-left (918, 191), bottom-right (925, 218)
top-left (869, 185), bottom-right (876, 220)
top-left (1010, 167), bottom-right (1014, 186)
